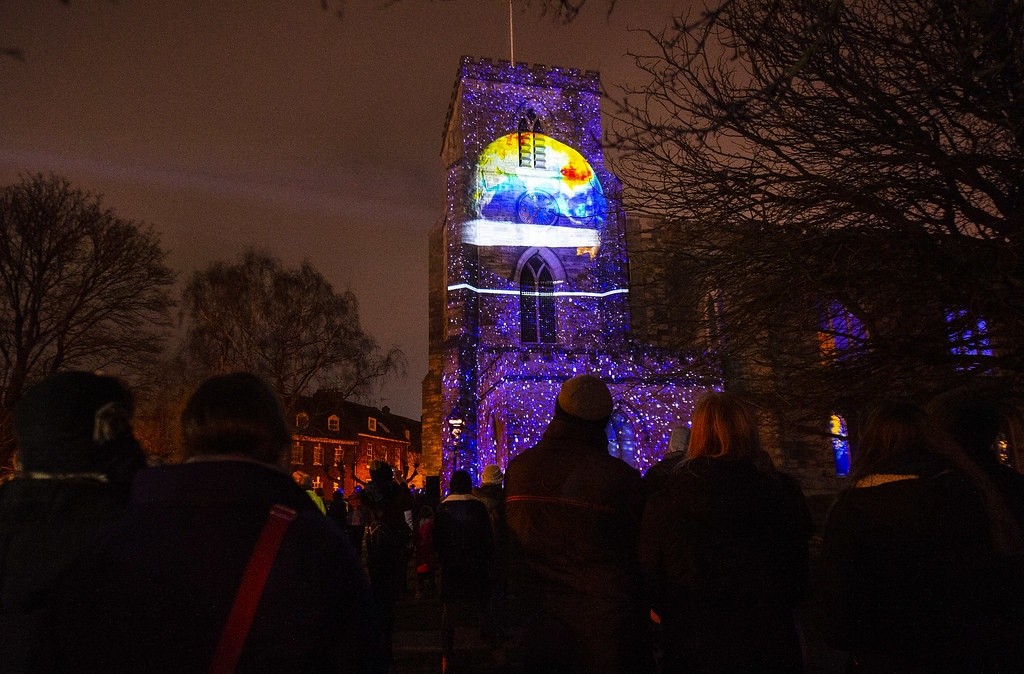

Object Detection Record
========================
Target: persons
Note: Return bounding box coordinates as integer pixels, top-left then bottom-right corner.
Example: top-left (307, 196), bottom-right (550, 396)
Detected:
top-left (1, 372), bottom-right (1024, 673)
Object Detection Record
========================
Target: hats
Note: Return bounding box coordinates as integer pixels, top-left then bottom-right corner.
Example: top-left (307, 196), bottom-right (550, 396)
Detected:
top-left (481, 464), bottom-right (504, 486)
top-left (450, 470), bottom-right (472, 494)
top-left (670, 426), bottom-right (692, 453)
top-left (921, 376), bottom-right (999, 456)
top-left (555, 374), bottom-right (613, 430)
top-left (369, 460), bottom-right (392, 483)
